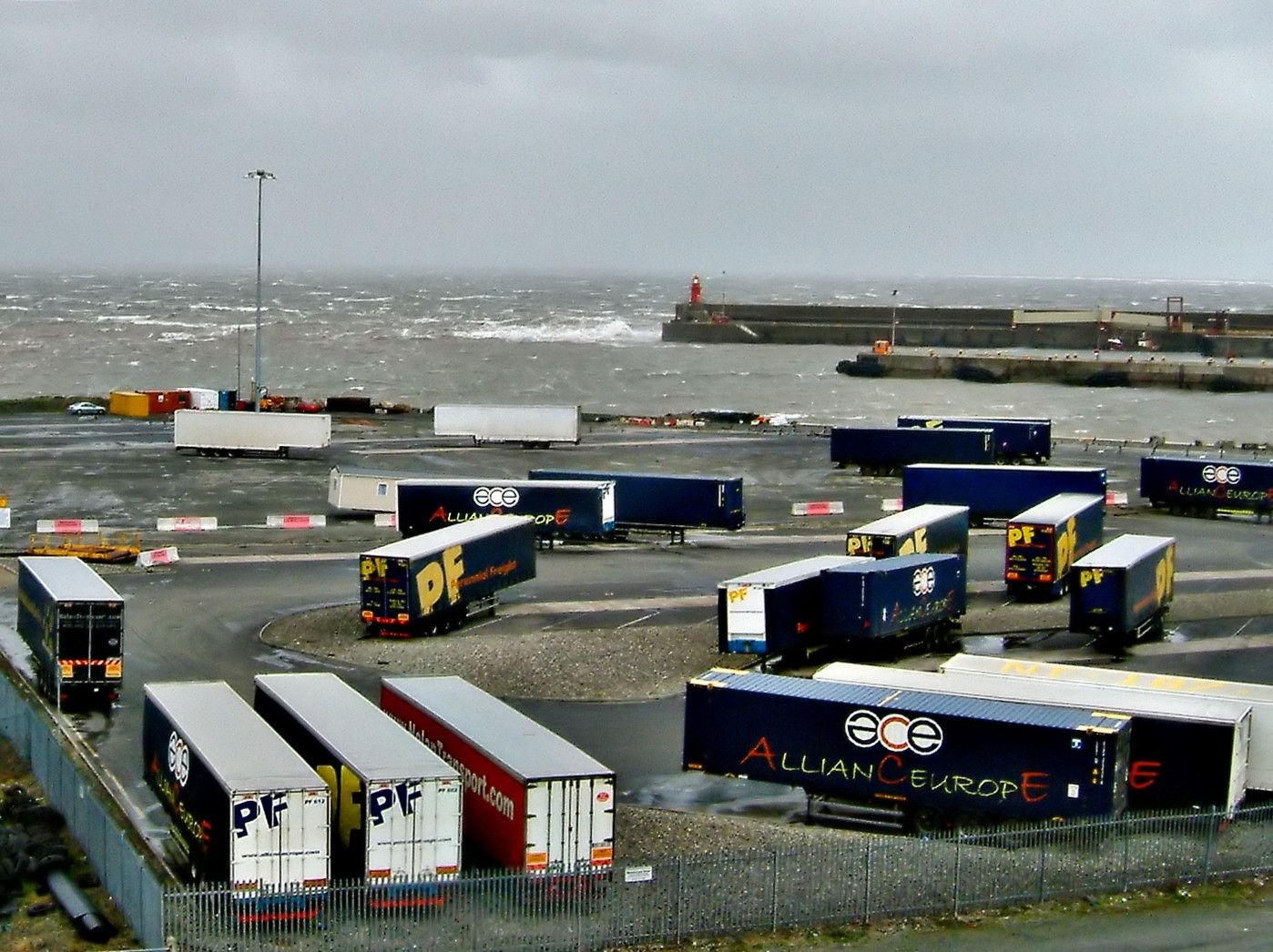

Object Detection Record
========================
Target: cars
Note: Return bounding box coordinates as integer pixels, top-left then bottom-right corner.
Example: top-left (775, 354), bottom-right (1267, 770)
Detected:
top-left (67, 401), bottom-right (107, 417)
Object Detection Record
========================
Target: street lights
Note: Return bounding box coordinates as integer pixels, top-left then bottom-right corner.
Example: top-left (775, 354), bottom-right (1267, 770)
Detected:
top-left (721, 271), bottom-right (726, 316)
top-left (244, 168), bottom-right (277, 412)
top-left (891, 290), bottom-right (898, 353)
